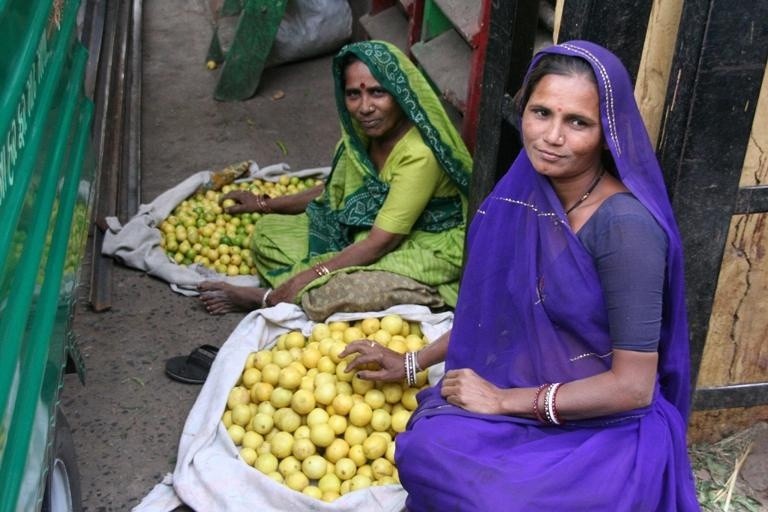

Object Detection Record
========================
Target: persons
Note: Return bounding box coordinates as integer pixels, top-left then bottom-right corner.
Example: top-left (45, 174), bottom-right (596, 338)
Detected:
top-left (193, 35), bottom-right (473, 320)
top-left (335, 35), bottom-right (704, 511)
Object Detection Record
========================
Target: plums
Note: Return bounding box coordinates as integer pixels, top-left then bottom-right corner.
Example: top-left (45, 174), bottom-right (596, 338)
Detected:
top-left (223, 312), bottom-right (430, 502)
top-left (157, 173), bottom-right (326, 277)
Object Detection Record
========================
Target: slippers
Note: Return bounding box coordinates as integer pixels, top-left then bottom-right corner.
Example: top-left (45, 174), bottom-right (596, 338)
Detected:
top-left (166, 343), bottom-right (219, 384)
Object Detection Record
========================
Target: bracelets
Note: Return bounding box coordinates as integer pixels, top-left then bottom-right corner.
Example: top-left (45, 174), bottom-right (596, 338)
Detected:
top-left (256, 192), bottom-right (272, 213)
top-left (406, 349), bottom-right (426, 389)
top-left (311, 260), bottom-right (331, 277)
top-left (535, 382), bottom-right (568, 427)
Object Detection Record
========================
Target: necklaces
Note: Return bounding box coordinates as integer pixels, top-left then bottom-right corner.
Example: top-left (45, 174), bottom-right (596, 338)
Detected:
top-left (544, 168), bottom-right (607, 232)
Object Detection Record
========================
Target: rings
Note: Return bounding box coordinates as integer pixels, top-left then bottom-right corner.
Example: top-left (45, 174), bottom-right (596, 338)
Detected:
top-left (370, 340), bottom-right (377, 348)
